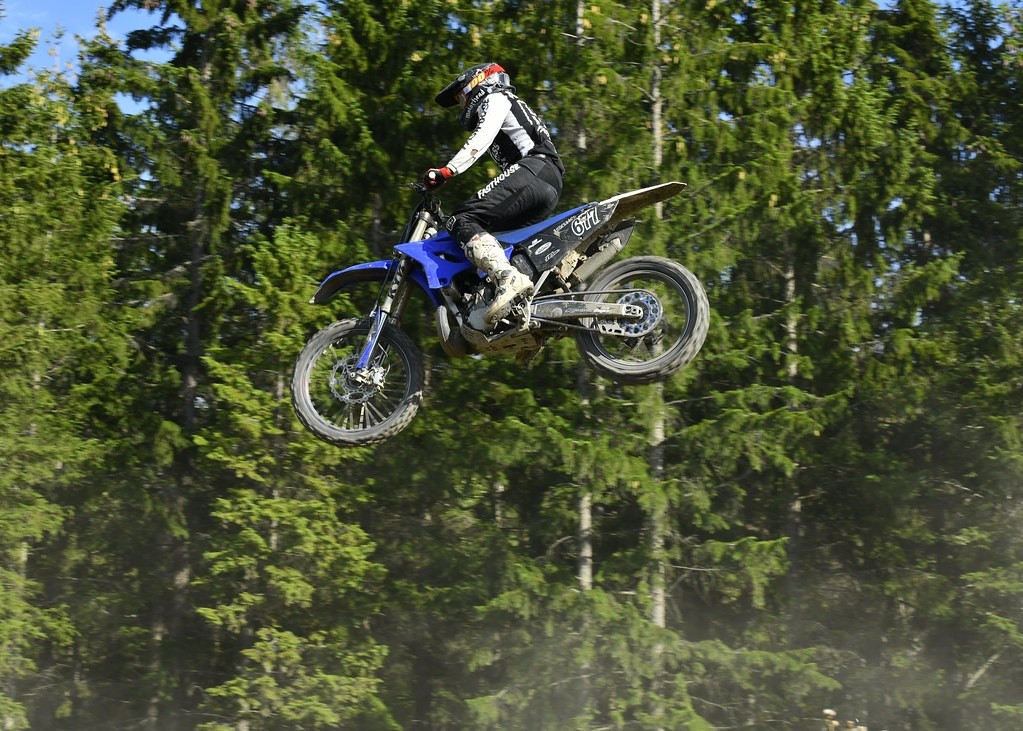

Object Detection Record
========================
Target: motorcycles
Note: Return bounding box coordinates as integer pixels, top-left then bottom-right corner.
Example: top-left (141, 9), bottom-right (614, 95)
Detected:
top-left (290, 173), bottom-right (711, 449)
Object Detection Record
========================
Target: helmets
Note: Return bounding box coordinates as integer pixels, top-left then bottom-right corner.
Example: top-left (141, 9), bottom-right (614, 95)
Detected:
top-left (435, 63), bottom-right (516, 132)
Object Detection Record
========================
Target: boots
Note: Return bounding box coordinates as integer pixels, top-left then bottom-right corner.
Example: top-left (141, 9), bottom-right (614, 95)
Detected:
top-left (463, 232), bottom-right (534, 324)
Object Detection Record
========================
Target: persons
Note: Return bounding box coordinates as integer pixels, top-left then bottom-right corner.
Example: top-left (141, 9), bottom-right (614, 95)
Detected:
top-left (423, 63), bottom-right (564, 324)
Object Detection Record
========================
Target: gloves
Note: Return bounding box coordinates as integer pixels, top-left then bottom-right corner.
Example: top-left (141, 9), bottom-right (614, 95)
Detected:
top-left (423, 167), bottom-right (453, 187)
